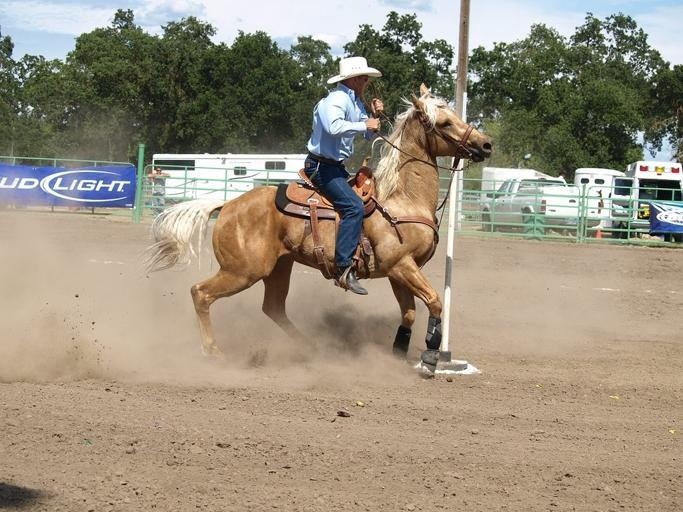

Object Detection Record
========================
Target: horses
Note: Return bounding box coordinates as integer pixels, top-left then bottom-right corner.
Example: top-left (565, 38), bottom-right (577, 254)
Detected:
top-left (138, 82), bottom-right (493, 378)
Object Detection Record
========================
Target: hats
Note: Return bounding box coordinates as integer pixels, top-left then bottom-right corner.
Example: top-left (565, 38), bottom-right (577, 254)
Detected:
top-left (326, 56), bottom-right (383, 84)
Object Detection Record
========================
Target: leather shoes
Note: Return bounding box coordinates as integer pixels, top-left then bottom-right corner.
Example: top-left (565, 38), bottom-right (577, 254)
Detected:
top-left (308, 152), bottom-right (342, 166)
top-left (334, 267), bottom-right (368, 295)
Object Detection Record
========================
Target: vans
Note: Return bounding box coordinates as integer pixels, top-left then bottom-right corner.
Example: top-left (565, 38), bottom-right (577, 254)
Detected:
top-left (610, 160), bottom-right (683, 242)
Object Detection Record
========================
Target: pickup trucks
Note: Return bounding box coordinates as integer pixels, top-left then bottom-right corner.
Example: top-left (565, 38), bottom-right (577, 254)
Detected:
top-left (480, 177), bottom-right (601, 237)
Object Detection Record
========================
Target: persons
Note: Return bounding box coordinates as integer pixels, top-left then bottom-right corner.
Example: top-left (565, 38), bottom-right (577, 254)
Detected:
top-left (147, 166), bottom-right (169, 222)
top-left (304, 56), bottom-right (386, 295)
